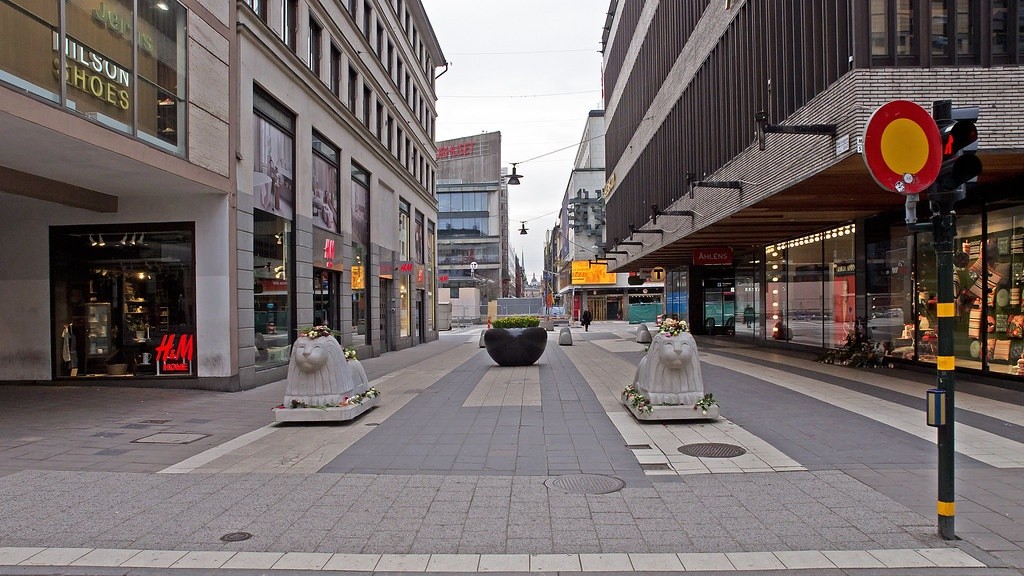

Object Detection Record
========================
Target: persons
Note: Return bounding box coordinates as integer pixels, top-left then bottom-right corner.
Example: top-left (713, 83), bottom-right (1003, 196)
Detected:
top-left (743, 304), bottom-right (755, 329)
top-left (581, 308), bottom-right (592, 332)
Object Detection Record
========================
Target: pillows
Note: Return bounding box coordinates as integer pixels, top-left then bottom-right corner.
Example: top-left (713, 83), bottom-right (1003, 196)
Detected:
top-left (980, 338), bottom-right (997, 360)
top-left (994, 340), bottom-right (1012, 361)
top-left (1006, 314), bottom-right (1023, 339)
top-left (994, 261), bottom-right (1013, 285)
top-left (969, 266), bottom-right (1001, 299)
top-left (969, 259), bottom-right (987, 275)
top-left (968, 309), bottom-right (982, 338)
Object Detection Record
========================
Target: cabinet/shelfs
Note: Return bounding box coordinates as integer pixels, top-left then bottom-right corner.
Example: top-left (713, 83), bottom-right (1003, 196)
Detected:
top-left (124, 292), bottom-right (149, 314)
top-left (82, 303), bottom-right (112, 363)
top-left (157, 306), bottom-right (170, 333)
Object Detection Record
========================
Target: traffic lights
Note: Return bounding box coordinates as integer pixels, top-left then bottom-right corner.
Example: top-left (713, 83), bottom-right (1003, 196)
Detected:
top-left (934, 100), bottom-right (983, 211)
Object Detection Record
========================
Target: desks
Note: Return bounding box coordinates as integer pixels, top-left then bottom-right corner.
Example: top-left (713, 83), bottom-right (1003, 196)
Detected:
top-left (908, 329), bottom-right (934, 352)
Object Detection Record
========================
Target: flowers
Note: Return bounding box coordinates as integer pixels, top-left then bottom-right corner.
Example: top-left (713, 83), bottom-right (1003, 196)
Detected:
top-left (128, 321), bottom-right (151, 331)
top-left (622, 318), bottom-right (720, 417)
top-left (293, 325), bottom-right (381, 408)
top-left (491, 316), bottom-right (541, 330)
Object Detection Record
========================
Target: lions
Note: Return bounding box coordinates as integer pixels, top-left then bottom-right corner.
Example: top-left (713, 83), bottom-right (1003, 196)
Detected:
top-left (632, 332), bottom-right (705, 404)
top-left (283, 334), bottom-right (370, 408)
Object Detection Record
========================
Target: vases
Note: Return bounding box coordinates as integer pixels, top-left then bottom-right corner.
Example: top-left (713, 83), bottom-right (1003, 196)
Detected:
top-left (136, 330), bottom-right (146, 339)
top-left (483, 328), bottom-right (547, 367)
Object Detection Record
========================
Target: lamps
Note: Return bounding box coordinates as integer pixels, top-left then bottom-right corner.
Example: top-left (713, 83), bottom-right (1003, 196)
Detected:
top-left (119, 261), bottom-right (159, 269)
top-left (274, 231), bottom-right (284, 279)
top-left (614, 238), bottom-right (643, 252)
top-left (649, 205), bottom-right (693, 225)
top-left (587, 260), bottom-right (608, 268)
top-left (98, 234), bottom-right (106, 246)
top-left (595, 255), bottom-right (617, 263)
top-left (155, 0), bottom-right (172, 12)
top-left (629, 224), bottom-right (663, 240)
top-left (601, 248), bottom-right (628, 255)
top-left (684, 169), bottom-right (743, 200)
top-left (88, 234), bottom-right (97, 246)
top-left (755, 112), bottom-right (835, 152)
top-left (120, 232), bottom-right (145, 246)
top-left (505, 162), bottom-right (529, 237)
